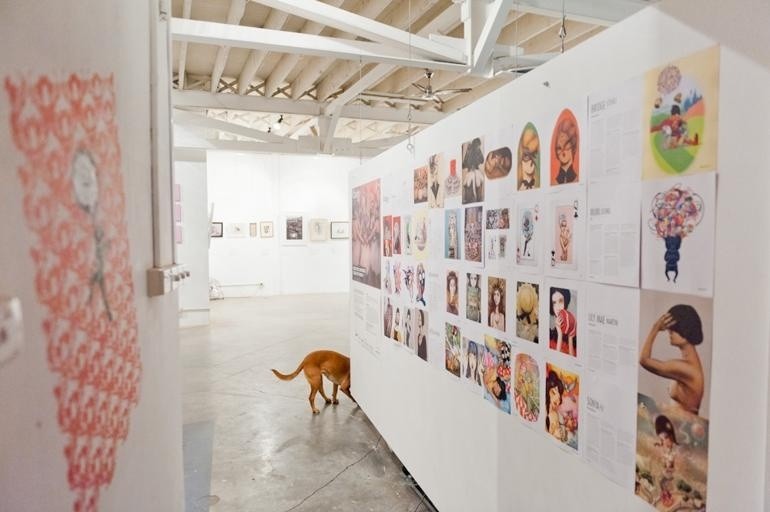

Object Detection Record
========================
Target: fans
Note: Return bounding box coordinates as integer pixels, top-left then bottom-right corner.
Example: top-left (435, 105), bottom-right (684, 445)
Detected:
top-left (413, 68), bottom-right (473, 104)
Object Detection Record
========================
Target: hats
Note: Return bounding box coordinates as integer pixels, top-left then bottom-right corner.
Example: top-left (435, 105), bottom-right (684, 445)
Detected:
top-left (516, 283), bottom-right (539, 327)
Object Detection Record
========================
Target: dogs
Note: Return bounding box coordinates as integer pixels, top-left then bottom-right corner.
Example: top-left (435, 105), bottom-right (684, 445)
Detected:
top-left (271, 350), bottom-right (359, 413)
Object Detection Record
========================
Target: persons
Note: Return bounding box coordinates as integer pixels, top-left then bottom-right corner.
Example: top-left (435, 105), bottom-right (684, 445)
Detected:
top-left (640, 302), bottom-right (705, 416)
top-left (662, 105), bottom-right (699, 149)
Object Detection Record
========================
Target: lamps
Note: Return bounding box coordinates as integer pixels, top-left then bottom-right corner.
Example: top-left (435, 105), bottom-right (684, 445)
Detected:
top-left (273, 116), bottom-right (283, 129)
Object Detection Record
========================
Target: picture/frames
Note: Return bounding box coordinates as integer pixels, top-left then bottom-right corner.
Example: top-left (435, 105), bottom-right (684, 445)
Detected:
top-left (260, 222), bottom-right (273, 237)
top-left (250, 223), bottom-right (256, 237)
top-left (331, 221), bottom-right (350, 239)
top-left (211, 222), bottom-right (223, 237)
